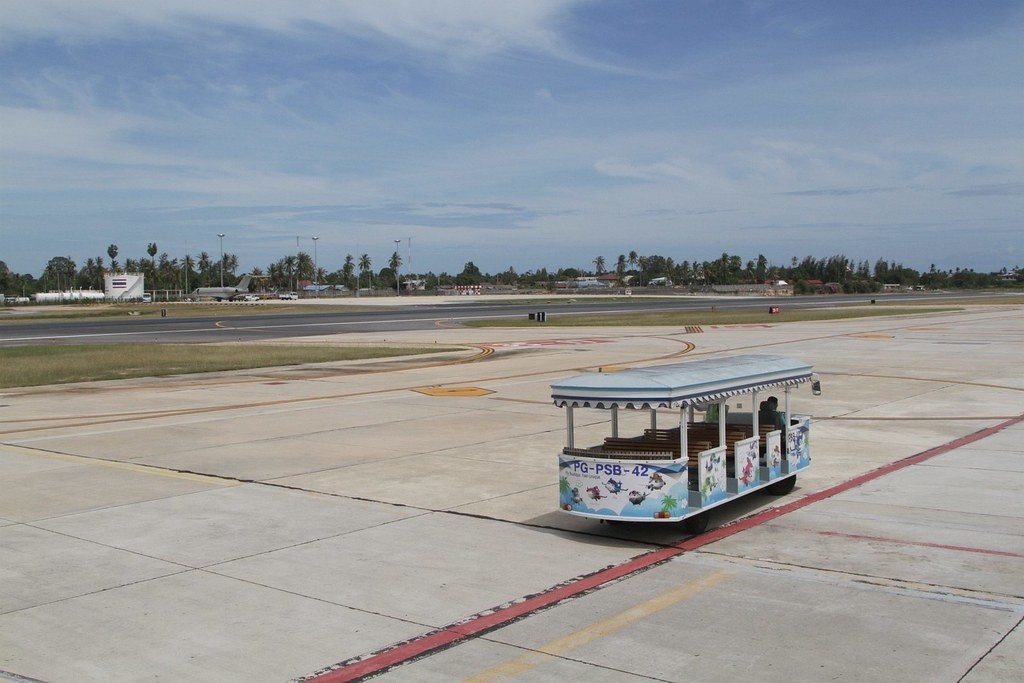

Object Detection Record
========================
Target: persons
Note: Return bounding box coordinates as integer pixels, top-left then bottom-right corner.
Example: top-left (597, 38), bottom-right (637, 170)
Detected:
top-left (767, 396), bottom-right (786, 427)
top-left (701, 402), bottom-right (729, 423)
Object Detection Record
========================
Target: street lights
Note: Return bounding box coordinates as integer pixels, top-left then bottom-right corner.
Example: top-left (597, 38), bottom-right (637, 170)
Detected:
top-left (215, 233), bottom-right (225, 287)
top-left (311, 236), bottom-right (319, 298)
top-left (393, 239), bottom-right (402, 295)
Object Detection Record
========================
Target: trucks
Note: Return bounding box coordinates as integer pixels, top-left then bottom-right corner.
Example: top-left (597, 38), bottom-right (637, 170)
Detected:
top-left (279, 292), bottom-right (298, 301)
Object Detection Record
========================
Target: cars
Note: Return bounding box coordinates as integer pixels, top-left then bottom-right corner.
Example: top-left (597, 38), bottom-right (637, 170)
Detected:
top-left (245, 295), bottom-right (260, 301)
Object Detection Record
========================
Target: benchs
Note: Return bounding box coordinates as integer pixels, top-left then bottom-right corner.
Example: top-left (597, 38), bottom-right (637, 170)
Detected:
top-left (602, 421), bottom-right (779, 491)
